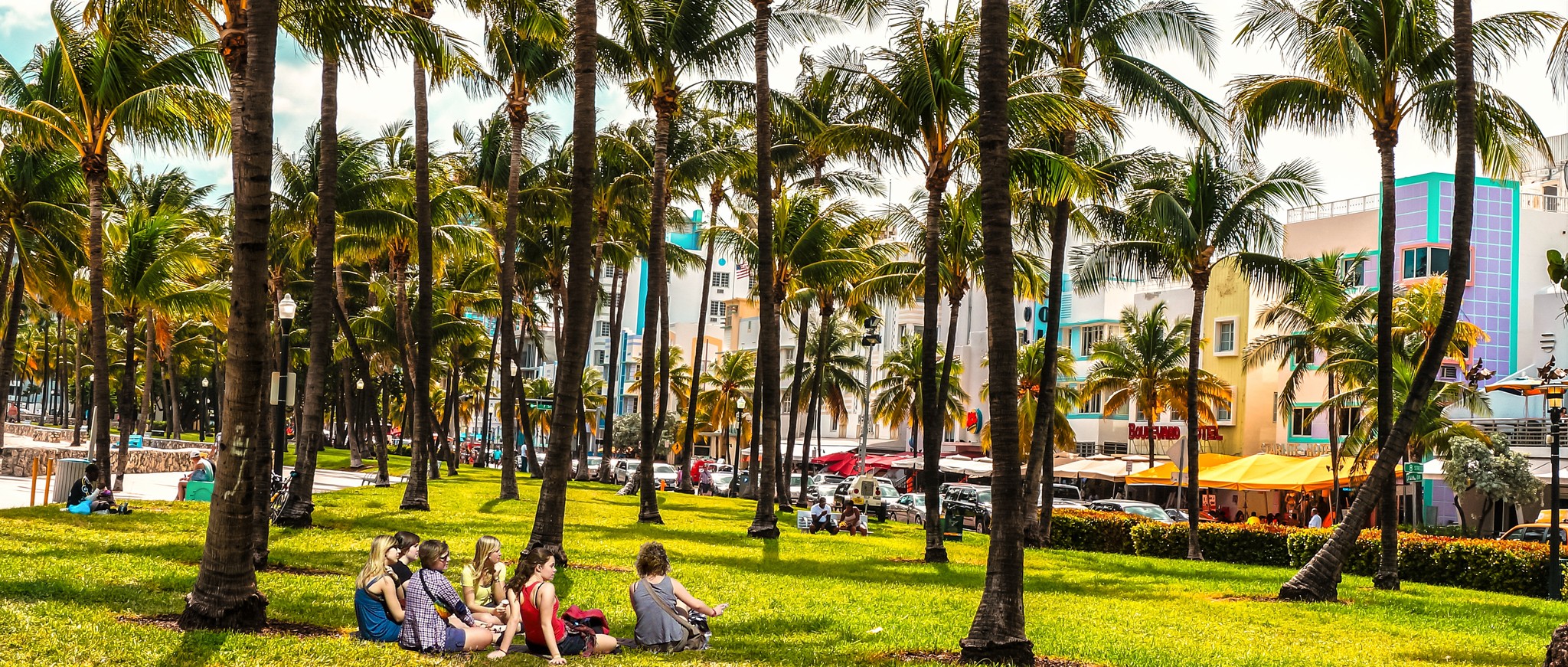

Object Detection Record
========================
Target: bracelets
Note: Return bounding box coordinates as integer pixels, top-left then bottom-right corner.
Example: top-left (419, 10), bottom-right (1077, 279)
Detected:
top-left (498, 648), bottom-right (507, 655)
top-left (394, 578), bottom-right (400, 588)
top-left (493, 576), bottom-right (499, 581)
top-left (494, 580), bottom-right (499, 582)
top-left (552, 654), bottom-right (561, 658)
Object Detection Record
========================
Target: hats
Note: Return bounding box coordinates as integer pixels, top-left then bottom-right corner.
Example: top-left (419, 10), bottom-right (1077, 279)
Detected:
top-left (200, 452), bottom-right (208, 459)
top-left (190, 450), bottom-right (199, 459)
top-left (85, 464), bottom-right (99, 472)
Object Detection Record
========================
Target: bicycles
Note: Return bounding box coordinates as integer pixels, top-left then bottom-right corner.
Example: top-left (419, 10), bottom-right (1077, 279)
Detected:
top-left (268, 468), bottom-right (300, 525)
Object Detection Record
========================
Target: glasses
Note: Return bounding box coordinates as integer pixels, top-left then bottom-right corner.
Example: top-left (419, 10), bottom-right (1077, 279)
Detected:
top-left (439, 551), bottom-right (449, 561)
top-left (386, 545), bottom-right (401, 551)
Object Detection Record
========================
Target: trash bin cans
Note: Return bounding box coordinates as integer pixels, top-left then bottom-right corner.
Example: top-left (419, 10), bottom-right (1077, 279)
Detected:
top-left (940, 515), bottom-right (964, 542)
top-left (52, 458), bottom-right (91, 502)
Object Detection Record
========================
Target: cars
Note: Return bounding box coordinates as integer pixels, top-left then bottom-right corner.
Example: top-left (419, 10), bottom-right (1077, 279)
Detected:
top-left (514, 456), bottom-right (523, 470)
top-left (36, 408), bottom-right (65, 417)
top-left (331, 434), bottom-right (349, 446)
top-left (526, 452), bottom-right (992, 537)
top-left (19, 408), bottom-right (32, 413)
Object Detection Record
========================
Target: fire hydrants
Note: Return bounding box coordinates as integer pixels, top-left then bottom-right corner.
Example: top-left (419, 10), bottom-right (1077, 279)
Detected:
top-left (659, 480), bottom-right (667, 491)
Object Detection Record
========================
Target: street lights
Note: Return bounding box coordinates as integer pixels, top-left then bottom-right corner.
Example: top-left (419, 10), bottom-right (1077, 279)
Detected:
top-left (486, 405), bottom-right (494, 468)
top-left (28, 391), bottom-right (30, 411)
top-left (355, 379), bottom-right (365, 445)
top-left (206, 398), bottom-right (211, 412)
top-left (202, 377), bottom-right (210, 440)
top-left (87, 373), bottom-right (95, 432)
top-left (290, 416), bottom-right (293, 429)
top-left (730, 396), bottom-right (746, 497)
top-left (1532, 382), bottom-right (1568, 600)
top-left (859, 315), bottom-right (882, 475)
top-left (195, 399), bottom-right (200, 432)
top-left (49, 390), bottom-right (54, 415)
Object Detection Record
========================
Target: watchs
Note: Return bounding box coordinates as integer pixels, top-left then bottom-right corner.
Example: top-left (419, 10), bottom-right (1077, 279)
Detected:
top-left (711, 608), bottom-right (716, 617)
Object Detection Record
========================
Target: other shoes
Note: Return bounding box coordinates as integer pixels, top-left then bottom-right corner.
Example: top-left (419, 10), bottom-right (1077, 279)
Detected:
top-left (496, 631), bottom-right (505, 647)
top-left (515, 622), bottom-right (525, 634)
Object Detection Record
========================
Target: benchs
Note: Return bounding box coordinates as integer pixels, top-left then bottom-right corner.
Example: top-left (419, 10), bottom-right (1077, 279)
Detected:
top-left (426, 461), bottom-right (442, 476)
top-left (360, 468), bottom-right (379, 487)
top-left (796, 510), bottom-right (874, 536)
top-left (111, 434), bottom-right (142, 448)
top-left (184, 480), bottom-right (214, 502)
top-left (151, 430), bottom-right (166, 436)
top-left (400, 468), bottom-right (411, 483)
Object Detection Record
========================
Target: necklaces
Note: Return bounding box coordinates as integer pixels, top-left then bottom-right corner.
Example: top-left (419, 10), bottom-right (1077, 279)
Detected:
top-left (483, 573), bottom-right (492, 603)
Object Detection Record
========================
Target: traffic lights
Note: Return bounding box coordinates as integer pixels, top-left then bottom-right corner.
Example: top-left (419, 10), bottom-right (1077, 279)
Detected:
top-left (530, 404), bottom-right (534, 409)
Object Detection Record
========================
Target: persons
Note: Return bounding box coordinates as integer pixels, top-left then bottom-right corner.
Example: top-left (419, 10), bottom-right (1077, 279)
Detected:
top-left (66, 464), bottom-right (132, 516)
top-left (8, 402), bottom-right (18, 423)
top-left (460, 439), bottom-right (529, 472)
top-left (809, 490), bottom-right (1335, 552)
top-left (699, 465), bottom-right (712, 496)
top-left (676, 467), bottom-right (683, 488)
top-left (1559, 406), bottom-right (1568, 444)
top-left (173, 450), bottom-right (216, 501)
top-left (354, 532), bottom-right (729, 664)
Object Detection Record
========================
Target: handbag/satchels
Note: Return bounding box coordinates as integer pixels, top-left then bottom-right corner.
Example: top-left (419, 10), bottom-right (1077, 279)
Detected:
top-left (190, 469), bottom-right (208, 481)
top-left (433, 600), bottom-right (453, 628)
top-left (383, 599), bottom-right (406, 624)
top-left (68, 500), bottom-right (93, 515)
top-left (561, 605), bottom-right (609, 634)
top-left (685, 620), bottom-right (712, 650)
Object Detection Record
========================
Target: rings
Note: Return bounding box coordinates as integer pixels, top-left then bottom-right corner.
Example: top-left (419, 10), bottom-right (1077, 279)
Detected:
top-left (722, 610), bottom-right (724, 612)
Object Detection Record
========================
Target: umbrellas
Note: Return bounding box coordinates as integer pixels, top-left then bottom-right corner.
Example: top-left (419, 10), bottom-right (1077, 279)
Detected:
top-left (809, 449), bottom-right (1405, 525)
top-left (1484, 371), bottom-right (1568, 418)
top-left (433, 433), bottom-right (454, 442)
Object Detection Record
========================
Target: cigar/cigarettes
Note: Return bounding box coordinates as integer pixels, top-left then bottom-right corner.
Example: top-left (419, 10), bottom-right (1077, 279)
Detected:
top-left (724, 603), bottom-right (728, 606)
